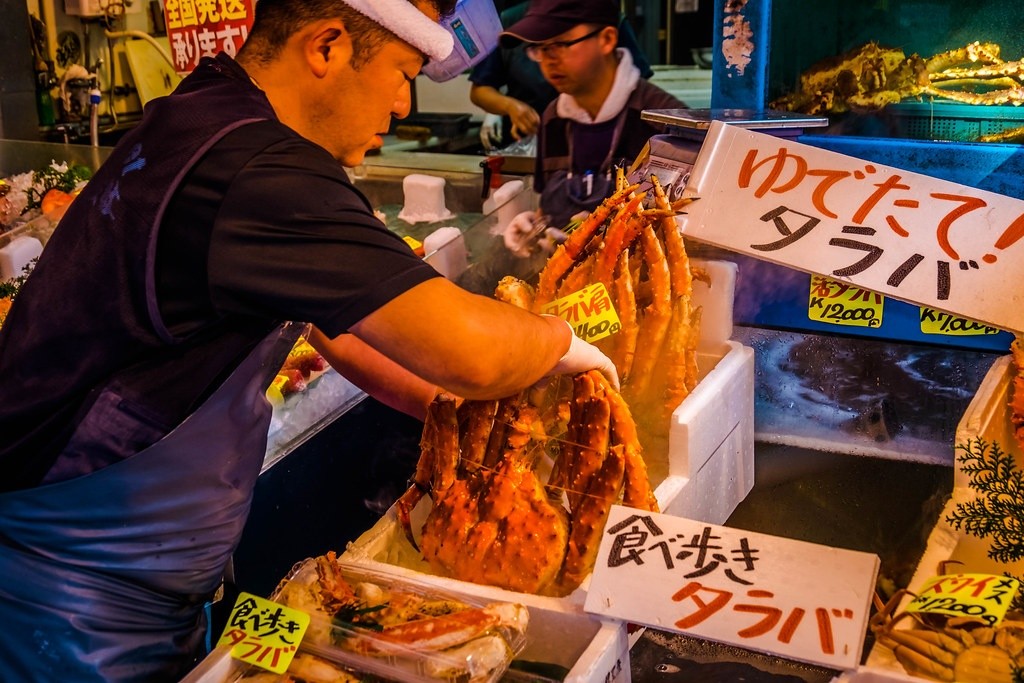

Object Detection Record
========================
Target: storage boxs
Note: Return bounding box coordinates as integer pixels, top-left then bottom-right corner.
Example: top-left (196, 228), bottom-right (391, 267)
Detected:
top-left (184, 327), bottom-right (752, 683)
top-left (713, 0), bottom-right (1024, 147)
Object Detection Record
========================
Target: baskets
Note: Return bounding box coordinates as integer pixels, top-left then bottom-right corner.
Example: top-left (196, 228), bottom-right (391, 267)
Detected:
top-left (888, 97), bottom-right (1024, 141)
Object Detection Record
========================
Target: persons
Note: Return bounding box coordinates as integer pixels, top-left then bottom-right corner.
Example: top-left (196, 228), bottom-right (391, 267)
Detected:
top-left (0, 0), bottom-right (621, 683)
top-left (497, 0), bottom-right (691, 257)
top-left (467, 0), bottom-right (654, 154)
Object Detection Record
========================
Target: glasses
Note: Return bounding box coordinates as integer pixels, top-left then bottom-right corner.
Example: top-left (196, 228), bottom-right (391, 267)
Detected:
top-left (522, 26), bottom-right (603, 62)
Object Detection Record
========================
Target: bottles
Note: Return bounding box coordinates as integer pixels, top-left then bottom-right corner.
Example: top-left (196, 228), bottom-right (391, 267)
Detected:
top-left (37, 61), bottom-right (61, 126)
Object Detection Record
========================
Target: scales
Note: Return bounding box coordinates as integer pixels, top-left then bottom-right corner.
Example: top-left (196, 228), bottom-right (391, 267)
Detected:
top-left (618, 107), bottom-right (830, 240)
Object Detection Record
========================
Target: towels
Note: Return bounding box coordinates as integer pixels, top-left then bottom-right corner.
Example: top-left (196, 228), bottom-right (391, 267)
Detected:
top-left (342, 0), bottom-right (454, 61)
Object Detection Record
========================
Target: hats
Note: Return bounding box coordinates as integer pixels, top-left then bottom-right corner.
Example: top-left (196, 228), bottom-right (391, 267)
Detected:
top-left (497, 0), bottom-right (621, 48)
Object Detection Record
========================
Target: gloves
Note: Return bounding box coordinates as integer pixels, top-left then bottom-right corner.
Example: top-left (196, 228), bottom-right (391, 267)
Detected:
top-left (504, 210), bottom-right (540, 258)
top-left (540, 313), bottom-right (620, 394)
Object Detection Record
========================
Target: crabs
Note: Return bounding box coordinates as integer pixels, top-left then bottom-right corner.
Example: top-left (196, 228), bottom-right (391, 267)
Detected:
top-left (223, 547), bottom-right (531, 683)
top-left (766, 37), bottom-right (1024, 144)
top-left (868, 556), bottom-right (1024, 683)
top-left (315, 166), bottom-right (701, 673)
